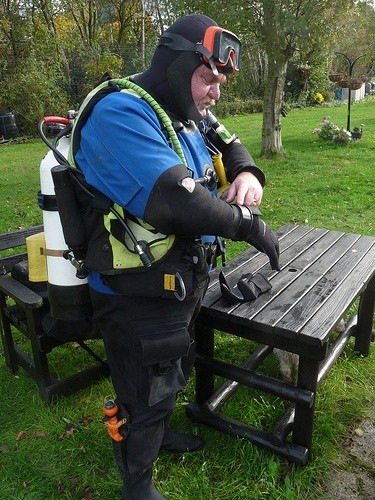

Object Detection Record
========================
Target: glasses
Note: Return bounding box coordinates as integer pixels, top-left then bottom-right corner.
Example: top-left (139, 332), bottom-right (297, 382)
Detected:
top-left (157, 26), bottom-right (242, 78)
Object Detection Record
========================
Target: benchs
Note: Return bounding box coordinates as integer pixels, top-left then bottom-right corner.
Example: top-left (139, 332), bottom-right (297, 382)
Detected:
top-left (186, 224), bottom-right (375, 465)
top-left (0, 225), bottom-right (111, 406)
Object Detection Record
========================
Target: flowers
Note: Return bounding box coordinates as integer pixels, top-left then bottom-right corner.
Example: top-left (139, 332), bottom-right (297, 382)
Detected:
top-left (332, 125), bottom-right (352, 143)
top-left (311, 116), bottom-right (340, 138)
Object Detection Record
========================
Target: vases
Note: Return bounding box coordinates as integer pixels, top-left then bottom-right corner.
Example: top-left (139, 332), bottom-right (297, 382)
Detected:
top-left (321, 134), bottom-right (333, 141)
top-left (334, 138), bottom-right (345, 144)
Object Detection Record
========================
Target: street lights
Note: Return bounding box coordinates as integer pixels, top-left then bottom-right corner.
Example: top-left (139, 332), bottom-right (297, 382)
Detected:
top-left (328, 51), bottom-right (369, 138)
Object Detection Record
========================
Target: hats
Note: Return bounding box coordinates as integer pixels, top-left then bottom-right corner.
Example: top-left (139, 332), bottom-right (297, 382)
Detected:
top-left (134, 14), bottom-right (241, 121)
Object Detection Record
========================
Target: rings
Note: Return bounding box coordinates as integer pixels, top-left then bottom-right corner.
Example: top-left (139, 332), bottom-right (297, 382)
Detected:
top-left (253, 199), bottom-right (256, 202)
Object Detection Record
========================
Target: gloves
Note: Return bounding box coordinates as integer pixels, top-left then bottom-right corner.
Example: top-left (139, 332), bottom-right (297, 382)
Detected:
top-left (219, 271), bottom-right (272, 301)
top-left (224, 205), bottom-right (281, 271)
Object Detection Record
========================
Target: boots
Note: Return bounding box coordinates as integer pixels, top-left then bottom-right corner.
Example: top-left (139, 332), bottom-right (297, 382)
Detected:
top-left (102, 398), bottom-right (204, 500)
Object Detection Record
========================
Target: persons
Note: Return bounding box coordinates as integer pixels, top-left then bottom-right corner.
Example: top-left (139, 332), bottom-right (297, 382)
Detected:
top-left (67, 14), bottom-right (281, 500)
